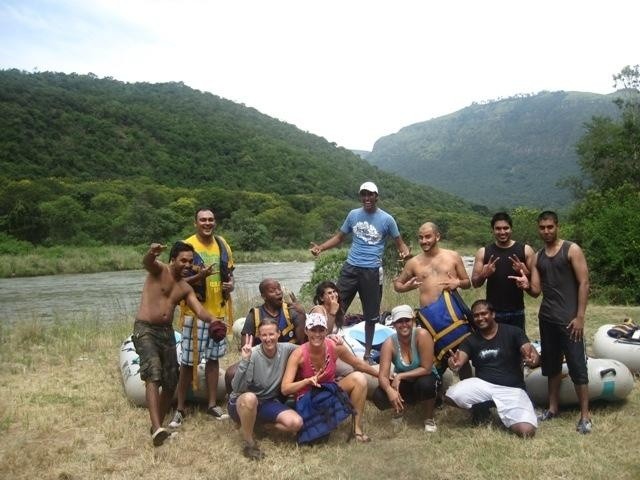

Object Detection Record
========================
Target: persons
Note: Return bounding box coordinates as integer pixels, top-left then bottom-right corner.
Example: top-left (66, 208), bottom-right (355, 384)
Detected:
top-left (310, 181), bottom-right (409, 365)
top-left (471, 212), bottom-right (535, 332)
top-left (168, 208), bottom-right (234, 428)
top-left (445, 301), bottom-right (540, 440)
top-left (225, 278), bottom-right (395, 461)
top-left (373, 305), bottom-right (442, 433)
top-left (506, 209), bottom-right (594, 434)
top-left (132, 241), bottom-right (230, 448)
top-left (395, 221), bottom-right (472, 410)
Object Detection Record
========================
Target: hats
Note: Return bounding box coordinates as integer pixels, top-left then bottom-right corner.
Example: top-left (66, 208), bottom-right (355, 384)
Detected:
top-left (304, 312), bottom-right (328, 331)
top-left (390, 304), bottom-right (415, 322)
top-left (358, 181), bottom-right (379, 195)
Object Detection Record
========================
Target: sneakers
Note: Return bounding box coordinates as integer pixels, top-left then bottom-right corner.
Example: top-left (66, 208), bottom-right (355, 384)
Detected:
top-left (363, 354), bottom-right (377, 365)
top-left (537, 409), bottom-right (558, 422)
top-left (152, 426), bottom-right (169, 446)
top-left (422, 418), bottom-right (438, 434)
top-left (206, 405), bottom-right (230, 421)
top-left (169, 408), bottom-right (185, 428)
top-left (242, 442), bottom-right (264, 460)
top-left (576, 419), bottom-right (593, 435)
top-left (391, 403), bottom-right (409, 423)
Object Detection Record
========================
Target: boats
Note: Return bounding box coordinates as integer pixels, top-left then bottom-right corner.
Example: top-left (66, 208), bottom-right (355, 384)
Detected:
top-left (119, 330), bottom-right (228, 410)
top-left (329, 323), bottom-right (453, 399)
top-left (522, 323), bottom-right (639, 406)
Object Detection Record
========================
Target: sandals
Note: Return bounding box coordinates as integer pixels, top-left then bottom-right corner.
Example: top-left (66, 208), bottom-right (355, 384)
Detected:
top-left (352, 430), bottom-right (371, 443)
